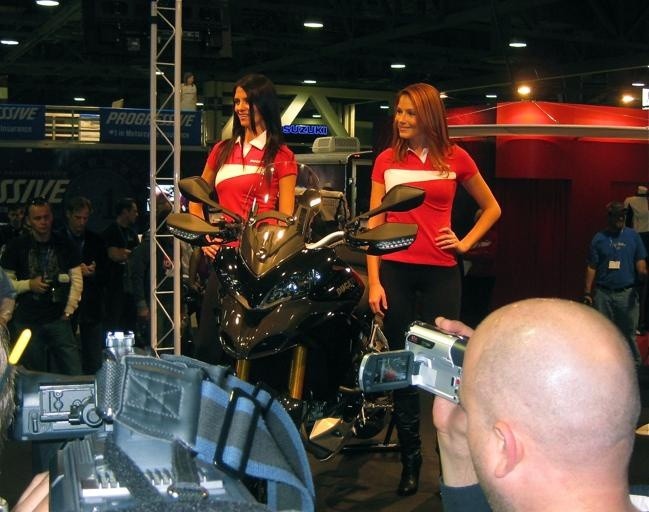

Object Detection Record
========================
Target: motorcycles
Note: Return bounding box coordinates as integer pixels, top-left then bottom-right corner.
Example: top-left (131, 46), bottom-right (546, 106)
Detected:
top-left (165, 160), bottom-right (427, 464)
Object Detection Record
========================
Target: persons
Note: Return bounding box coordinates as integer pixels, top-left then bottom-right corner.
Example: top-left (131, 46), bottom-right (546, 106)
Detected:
top-left (181, 72), bottom-right (197, 111)
top-left (620, 186), bottom-right (649, 335)
top-left (189, 74), bottom-right (299, 397)
top-left (0, 196), bottom-right (212, 376)
top-left (368, 83), bottom-right (502, 497)
top-left (433, 297), bottom-right (649, 512)
top-left (452, 208), bottom-right (493, 249)
top-left (584, 201), bottom-right (649, 371)
top-left (0, 324), bottom-right (50, 512)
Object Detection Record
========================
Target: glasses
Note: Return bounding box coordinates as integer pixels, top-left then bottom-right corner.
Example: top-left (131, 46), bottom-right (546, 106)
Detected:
top-left (28, 197), bottom-right (49, 206)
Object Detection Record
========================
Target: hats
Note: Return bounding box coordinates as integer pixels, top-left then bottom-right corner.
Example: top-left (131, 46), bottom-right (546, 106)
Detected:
top-left (605, 200), bottom-right (629, 217)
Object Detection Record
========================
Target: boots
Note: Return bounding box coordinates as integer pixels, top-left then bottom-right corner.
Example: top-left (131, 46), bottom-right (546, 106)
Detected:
top-left (388, 390), bottom-right (428, 499)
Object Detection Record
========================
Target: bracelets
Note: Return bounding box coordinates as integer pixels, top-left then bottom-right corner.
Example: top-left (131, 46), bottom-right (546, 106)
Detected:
top-left (585, 291), bottom-right (592, 296)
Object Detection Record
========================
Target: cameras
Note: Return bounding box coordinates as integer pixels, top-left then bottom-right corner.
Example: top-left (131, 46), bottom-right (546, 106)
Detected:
top-left (358, 320), bottom-right (468, 407)
